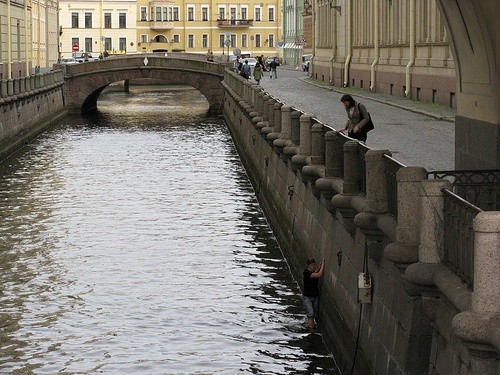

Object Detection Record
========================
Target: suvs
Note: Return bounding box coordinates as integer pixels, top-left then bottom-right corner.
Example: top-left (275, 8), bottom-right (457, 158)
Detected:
top-left (303, 54), bottom-right (315, 70)
top-left (76, 52), bottom-right (98, 63)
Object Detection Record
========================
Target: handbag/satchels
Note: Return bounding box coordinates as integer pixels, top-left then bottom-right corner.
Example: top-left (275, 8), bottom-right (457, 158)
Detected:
top-left (357, 103), bottom-right (375, 133)
top-left (240, 66), bottom-right (246, 78)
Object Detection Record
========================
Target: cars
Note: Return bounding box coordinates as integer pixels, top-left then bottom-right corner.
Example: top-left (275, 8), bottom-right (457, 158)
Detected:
top-left (242, 58), bottom-right (262, 70)
top-left (61, 57), bottom-right (79, 65)
top-left (265, 56), bottom-right (283, 65)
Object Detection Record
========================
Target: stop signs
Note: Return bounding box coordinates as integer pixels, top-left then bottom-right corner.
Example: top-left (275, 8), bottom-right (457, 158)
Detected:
top-left (73, 44), bottom-right (80, 52)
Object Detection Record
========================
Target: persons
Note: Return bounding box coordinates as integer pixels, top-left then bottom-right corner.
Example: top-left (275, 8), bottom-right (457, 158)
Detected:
top-left (253, 64), bottom-right (263, 85)
top-left (85, 53), bottom-right (88, 62)
top-left (241, 60), bottom-right (251, 79)
top-left (258, 55), bottom-right (268, 71)
top-left (301, 258), bottom-right (325, 328)
top-left (206, 49), bottom-right (214, 62)
top-left (234, 56), bottom-right (242, 75)
top-left (99, 51), bottom-right (109, 60)
top-left (269, 58), bottom-right (279, 79)
top-left (340, 94), bottom-right (369, 144)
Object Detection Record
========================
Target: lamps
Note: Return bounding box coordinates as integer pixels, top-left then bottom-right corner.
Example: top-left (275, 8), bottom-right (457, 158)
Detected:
top-left (303, 0), bottom-right (311, 16)
top-left (328, 0), bottom-right (341, 15)
